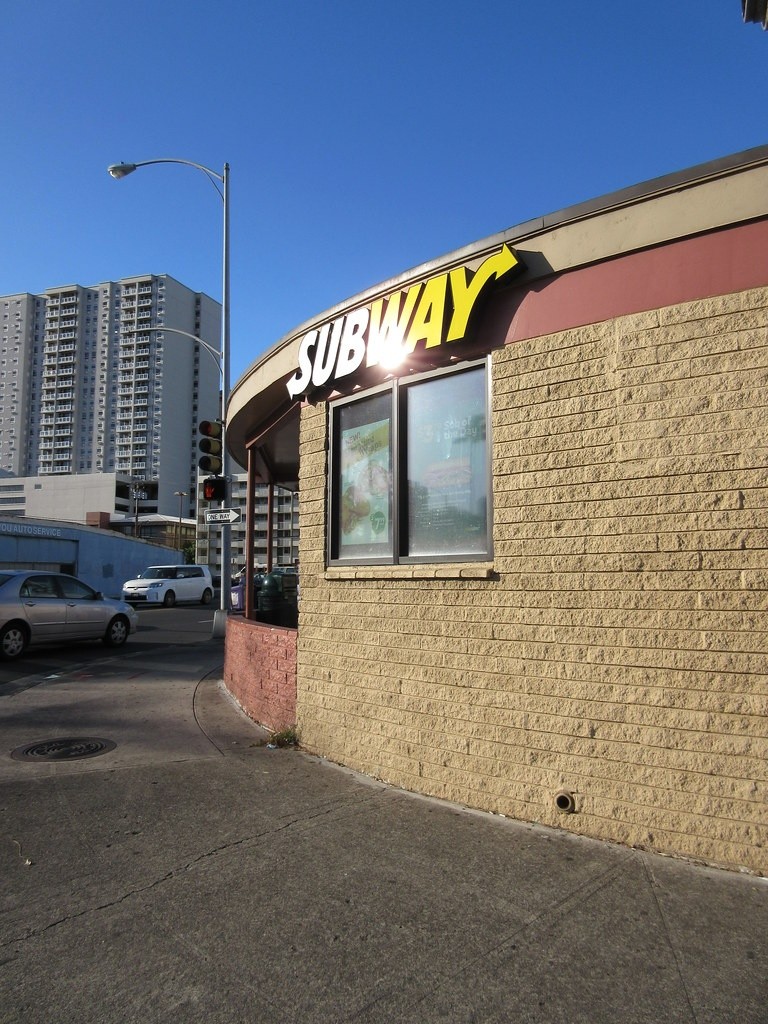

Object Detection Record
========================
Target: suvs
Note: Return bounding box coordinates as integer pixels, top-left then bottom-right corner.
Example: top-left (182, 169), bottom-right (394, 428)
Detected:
top-left (120, 564), bottom-right (216, 609)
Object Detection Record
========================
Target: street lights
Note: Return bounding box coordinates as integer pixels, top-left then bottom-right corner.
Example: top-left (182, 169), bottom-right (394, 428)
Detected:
top-left (108, 155), bottom-right (232, 610)
top-left (173, 491), bottom-right (189, 550)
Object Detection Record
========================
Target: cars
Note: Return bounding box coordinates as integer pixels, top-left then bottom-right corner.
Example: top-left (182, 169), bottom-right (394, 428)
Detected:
top-left (0, 570), bottom-right (140, 666)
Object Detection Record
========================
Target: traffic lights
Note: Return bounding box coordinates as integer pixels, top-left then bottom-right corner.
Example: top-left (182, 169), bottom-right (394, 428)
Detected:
top-left (198, 420), bottom-right (221, 475)
top-left (202, 477), bottom-right (225, 500)
